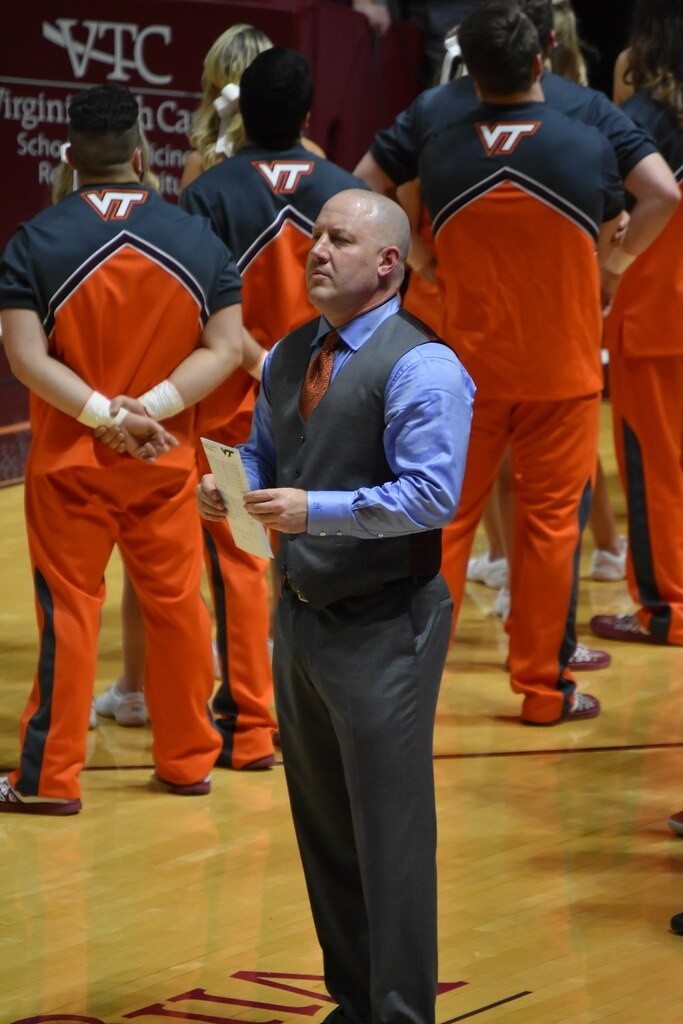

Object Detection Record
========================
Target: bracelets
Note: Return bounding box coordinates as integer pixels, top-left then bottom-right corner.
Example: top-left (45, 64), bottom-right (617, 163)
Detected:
top-left (76, 390), bottom-right (128, 431)
top-left (138, 380), bottom-right (184, 422)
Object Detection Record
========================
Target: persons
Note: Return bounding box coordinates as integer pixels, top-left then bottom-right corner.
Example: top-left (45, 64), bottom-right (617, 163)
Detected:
top-left (0, 83), bottom-right (244, 819)
top-left (190, 189), bottom-right (480, 1024)
top-left (53, 1), bottom-right (683, 772)
top-left (415, 4), bottom-right (629, 726)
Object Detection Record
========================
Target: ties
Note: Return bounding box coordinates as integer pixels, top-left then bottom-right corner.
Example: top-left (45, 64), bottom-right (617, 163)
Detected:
top-left (300, 333), bottom-right (337, 417)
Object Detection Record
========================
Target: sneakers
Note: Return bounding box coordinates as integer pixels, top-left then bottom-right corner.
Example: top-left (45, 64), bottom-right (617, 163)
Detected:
top-left (495, 586), bottom-right (511, 621)
top-left (591, 536), bottom-right (626, 580)
top-left (467, 552), bottom-right (507, 588)
top-left (568, 643), bottom-right (612, 670)
top-left (568, 691), bottom-right (601, 720)
top-left (169, 770), bottom-right (211, 795)
top-left (223, 743), bottom-right (276, 769)
top-left (590, 613), bottom-right (681, 644)
top-left (2, 773), bottom-right (82, 815)
top-left (91, 685), bottom-right (146, 726)
